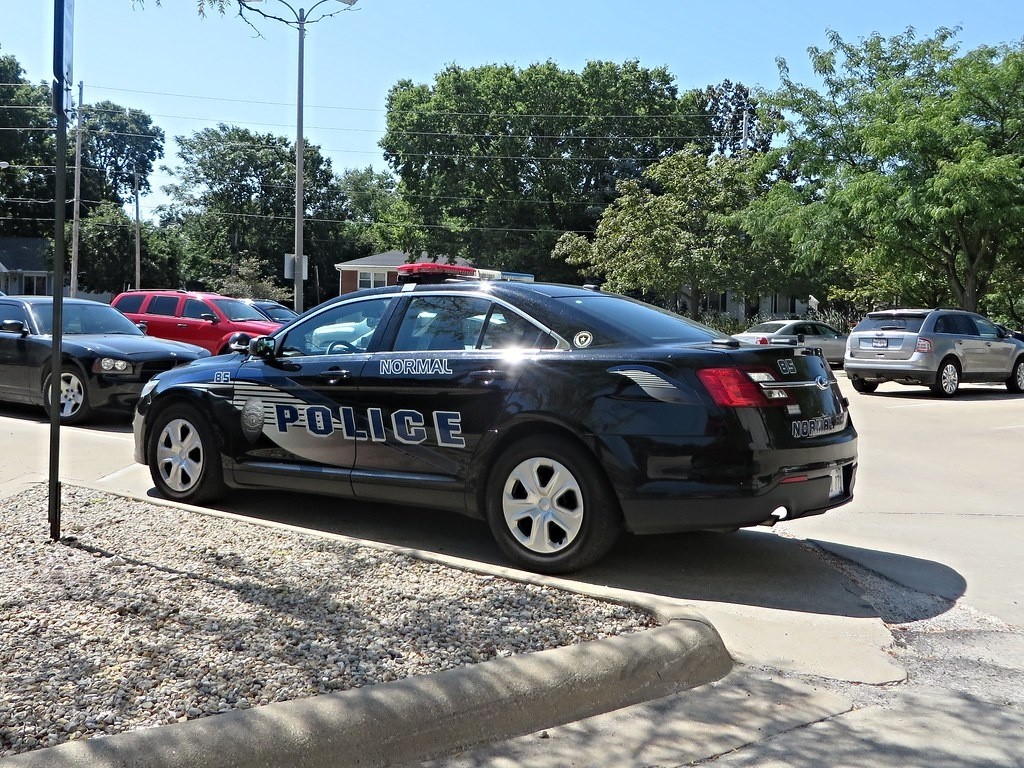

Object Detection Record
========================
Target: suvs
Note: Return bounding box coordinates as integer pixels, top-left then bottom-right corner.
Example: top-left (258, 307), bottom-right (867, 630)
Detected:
top-left (844, 305), bottom-right (1024, 398)
top-left (112, 289), bottom-right (283, 361)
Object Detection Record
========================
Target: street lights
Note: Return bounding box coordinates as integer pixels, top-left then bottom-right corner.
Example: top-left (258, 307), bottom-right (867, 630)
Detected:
top-left (276, 1), bottom-right (359, 315)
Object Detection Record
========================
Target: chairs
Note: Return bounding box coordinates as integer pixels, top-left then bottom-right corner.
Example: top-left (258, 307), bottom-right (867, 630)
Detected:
top-left (417, 314), bottom-right (464, 350)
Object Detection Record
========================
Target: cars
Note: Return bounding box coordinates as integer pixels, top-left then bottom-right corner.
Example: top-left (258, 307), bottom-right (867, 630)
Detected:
top-left (240, 296), bottom-right (303, 322)
top-left (127, 264), bottom-right (860, 576)
top-left (1, 293), bottom-right (212, 427)
top-left (731, 320), bottom-right (849, 364)
